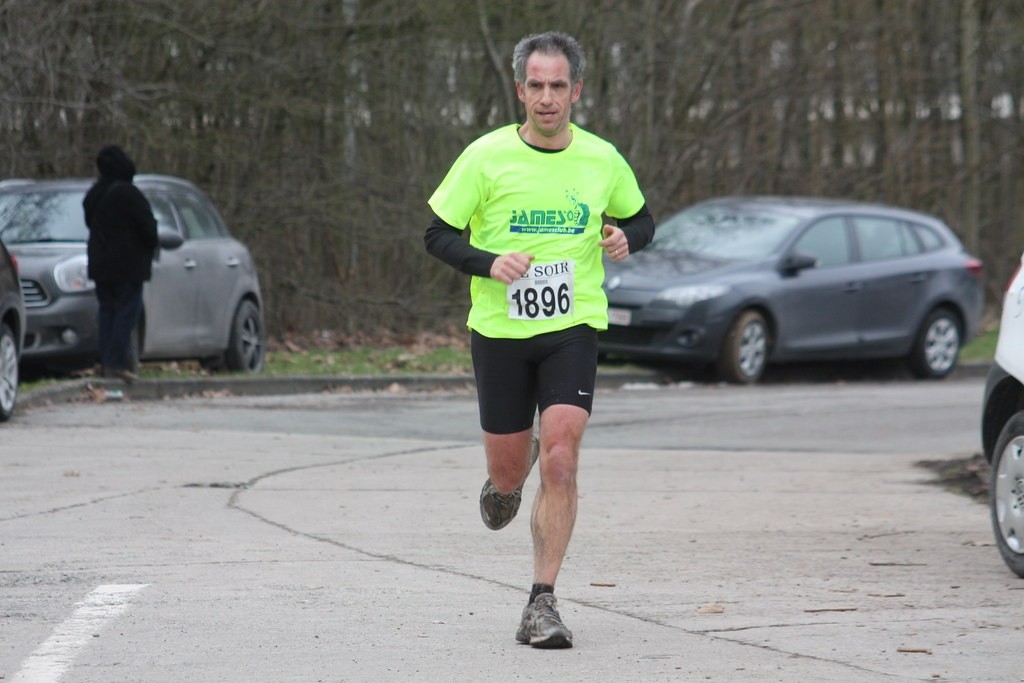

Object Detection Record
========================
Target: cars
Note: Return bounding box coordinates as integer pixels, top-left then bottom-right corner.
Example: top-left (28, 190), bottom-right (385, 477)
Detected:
top-left (0, 175), bottom-right (266, 377)
top-left (597, 194), bottom-right (986, 387)
top-left (0, 238), bottom-right (27, 423)
top-left (978, 259), bottom-right (1024, 579)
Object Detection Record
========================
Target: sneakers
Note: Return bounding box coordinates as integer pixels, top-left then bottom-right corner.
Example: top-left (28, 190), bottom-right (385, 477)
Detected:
top-left (516, 592), bottom-right (572, 648)
top-left (479, 435), bottom-right (539, 531)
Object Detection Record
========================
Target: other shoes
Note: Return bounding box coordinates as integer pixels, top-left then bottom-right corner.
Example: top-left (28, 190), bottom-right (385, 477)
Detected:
top-left (100, 366), bottom-right (139, 381)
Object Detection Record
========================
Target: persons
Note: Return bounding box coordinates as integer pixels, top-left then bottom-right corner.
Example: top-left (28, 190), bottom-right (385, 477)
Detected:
top-left (424, 31), bottom-right (655, 649)
top-left (81, 145), bottom-right (161, 382)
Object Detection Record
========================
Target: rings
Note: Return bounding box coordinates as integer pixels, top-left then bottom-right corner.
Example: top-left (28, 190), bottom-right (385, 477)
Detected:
top-left (616, 249), bottom-right (618, 256)
